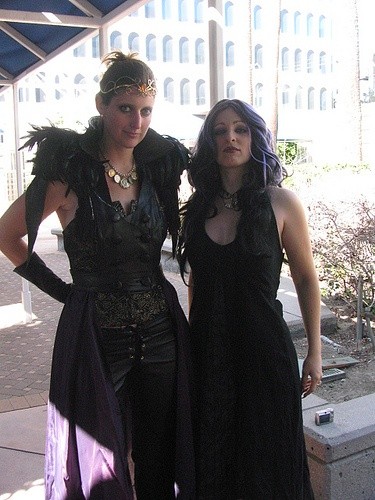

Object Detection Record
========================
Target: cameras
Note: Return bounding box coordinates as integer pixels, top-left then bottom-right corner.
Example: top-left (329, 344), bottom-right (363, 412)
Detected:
top-left (315, 408), bottom-right (334, 426)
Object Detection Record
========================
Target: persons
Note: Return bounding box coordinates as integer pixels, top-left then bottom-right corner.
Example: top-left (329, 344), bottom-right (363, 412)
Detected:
top-left (0, 52), bottom-right (199, 500)
top-left (184, 99), bottom-right (323, 500)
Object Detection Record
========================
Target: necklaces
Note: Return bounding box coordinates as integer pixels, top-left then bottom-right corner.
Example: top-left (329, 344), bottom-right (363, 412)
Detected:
top-left (98, 146), bottom-right (137, 190)
top-left (219, 185), bottom-right (240, 210)
